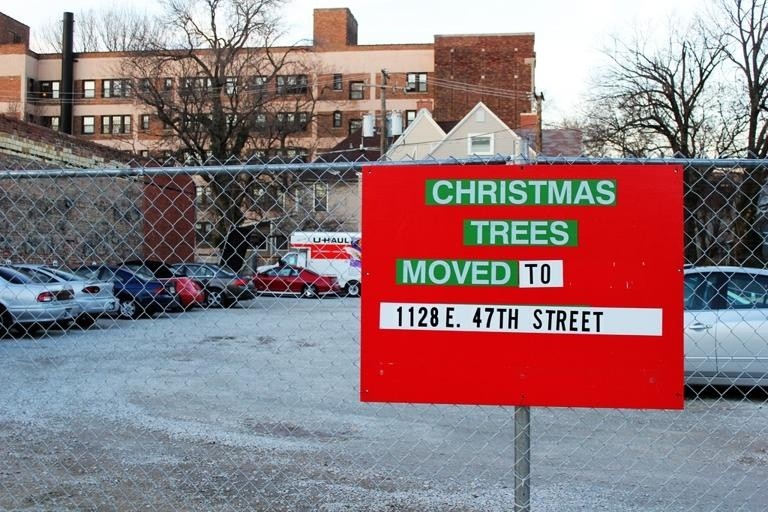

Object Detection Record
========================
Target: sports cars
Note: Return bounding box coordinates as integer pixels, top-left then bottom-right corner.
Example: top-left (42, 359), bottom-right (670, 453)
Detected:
top-left (115, 261), bottom-right (203, 312)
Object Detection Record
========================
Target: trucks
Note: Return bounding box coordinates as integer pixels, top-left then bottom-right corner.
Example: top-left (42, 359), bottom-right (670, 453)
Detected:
top-left (255, 231), bottom-right (361, 299)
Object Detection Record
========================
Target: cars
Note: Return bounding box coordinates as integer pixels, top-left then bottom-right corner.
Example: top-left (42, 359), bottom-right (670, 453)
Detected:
top-left (239, 266), bottom-right (338, 297)
top-left (172, 264), bottom-right (258, 307)
top-left (0, 267), bottom-right (76, 333)
top-left (13, 264), bottom-right (117, 327)
top-left (20, 294), bottom-right (40, 299)
top-left (69, 266), bottom-right (176, 319)
